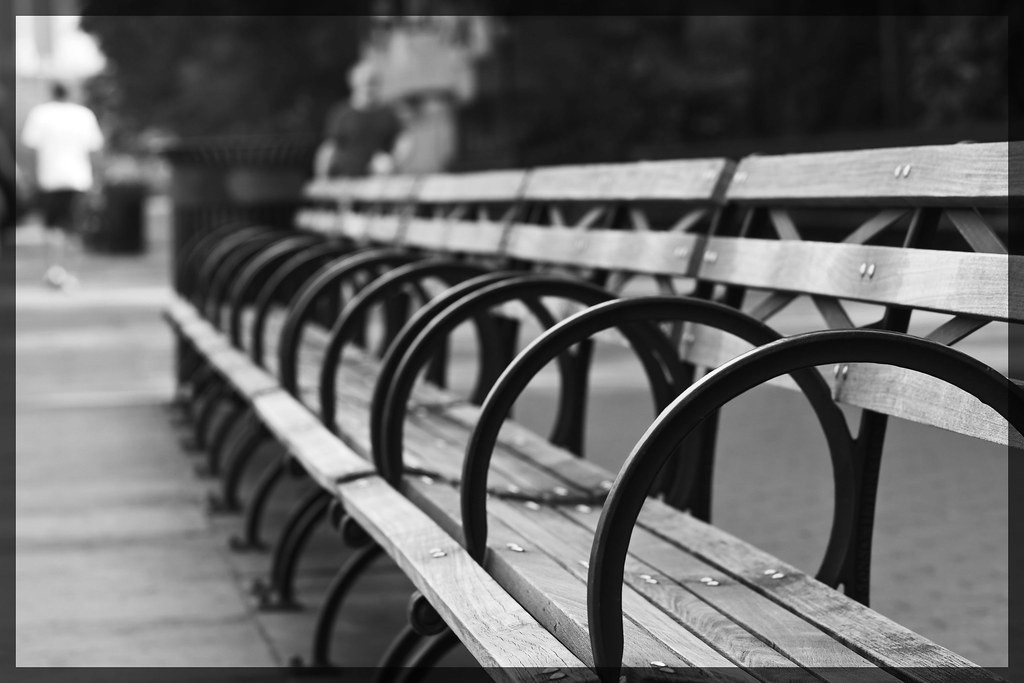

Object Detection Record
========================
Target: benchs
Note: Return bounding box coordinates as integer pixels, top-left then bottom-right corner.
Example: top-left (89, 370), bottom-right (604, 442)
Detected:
top-left (157, 140), bottom-right (1024, 683)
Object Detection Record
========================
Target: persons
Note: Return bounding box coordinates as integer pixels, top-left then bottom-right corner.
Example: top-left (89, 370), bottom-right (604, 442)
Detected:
top-left (311, 60), bottom-right (415, 176)
top-left (22, 83), bottom-right (104, 288)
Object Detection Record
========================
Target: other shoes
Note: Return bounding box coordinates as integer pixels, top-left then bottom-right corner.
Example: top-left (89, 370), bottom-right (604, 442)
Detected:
top-left (45, 266), bottom-right (75, 288)
